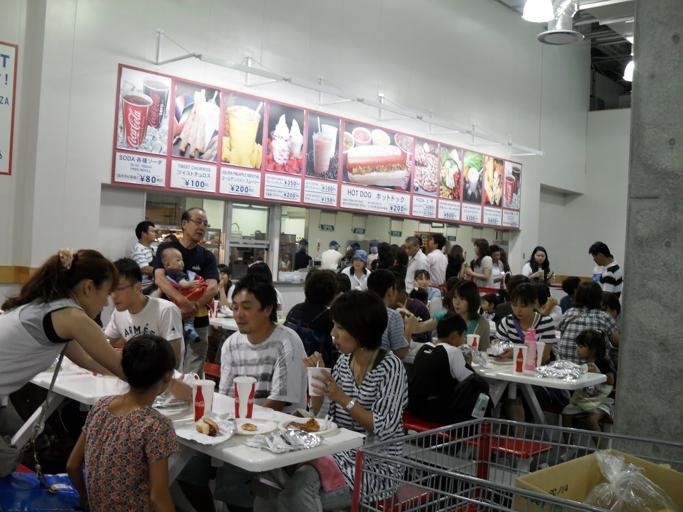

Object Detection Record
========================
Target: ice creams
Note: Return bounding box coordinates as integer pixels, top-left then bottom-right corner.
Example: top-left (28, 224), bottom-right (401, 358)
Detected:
top-left (290, 118), bottom-right (302, 156)
top-left (270, 114), bottom-right (292, 163)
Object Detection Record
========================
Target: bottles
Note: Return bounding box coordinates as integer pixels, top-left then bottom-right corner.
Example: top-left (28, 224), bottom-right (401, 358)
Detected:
top-left (234, 383), bottom-right (239, 418)
top-left (472, 337), bottom-right (477, 348)
top-left (516, 348), bottom-right (523, 372)
top-left (244, 383), bottom-right (254, 417)
top-left (524, 328), bottom-right (537, 368)
top-left (194, 384), bottom-right (204, 421)
top-left (182, 373), bottom-right (195, 387)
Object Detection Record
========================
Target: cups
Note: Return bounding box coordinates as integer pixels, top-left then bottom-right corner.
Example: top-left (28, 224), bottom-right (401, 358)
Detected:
top-left (512, 345), bottom-right (528, 373)
top-left (321, 124), bottom-right (337, 156)
top-left (209, 300), bottom-right (218, 318)
top-left (225, 105), bottom-right (260, 165)
top-left (342, 127), bottom-right (390, 154)
top-left (232, 377), bottom-right (257, 419)
top-left (466, 334), bottom-right (479, 349)
top-left (269, 130), bottom-right (303, 164)
top-left (311, 136), bottom-right (332, 176)
top-left (119, 77), bottom-right (168, 147)
top-left (192, 380), bottom-right (214, 422)
top-left (465, 167), bottom-right (480, 192)
top-left (538, 270), bottom-right (544, 280)
top-left (307, 367), bottom-right (332, 396)
top-left (505, 166), bottom-right (520, 205)
top-left (536, 342), bottom-right (545, 367)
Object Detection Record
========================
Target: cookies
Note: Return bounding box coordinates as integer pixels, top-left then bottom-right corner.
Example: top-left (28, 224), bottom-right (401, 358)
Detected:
top-left (241, 423), bottom-right (257, 432)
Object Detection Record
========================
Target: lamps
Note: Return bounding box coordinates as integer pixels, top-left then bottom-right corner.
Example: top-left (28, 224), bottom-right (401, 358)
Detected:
top-left (623, 36), bottom-right (635, 82)
top-left (521, 0), bottom-right (555, 24)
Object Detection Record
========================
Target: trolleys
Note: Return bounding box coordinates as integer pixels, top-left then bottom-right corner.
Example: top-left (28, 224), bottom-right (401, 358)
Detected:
top-left (353, 414), bottom-right (682, 511)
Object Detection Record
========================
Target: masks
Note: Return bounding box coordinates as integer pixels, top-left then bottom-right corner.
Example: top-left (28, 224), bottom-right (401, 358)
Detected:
top-left (370, 246), bottom-right (378, 253)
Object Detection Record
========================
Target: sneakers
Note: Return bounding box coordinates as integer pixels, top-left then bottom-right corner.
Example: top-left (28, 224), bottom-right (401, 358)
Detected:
top-left (559, 443), bottom-right (580, 461)
top-left (515, 457), bottom-right (536, 472)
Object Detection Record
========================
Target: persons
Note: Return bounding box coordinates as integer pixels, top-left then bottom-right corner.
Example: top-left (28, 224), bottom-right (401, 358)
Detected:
top-left (0, 246), bottom-right (197, 481)
top-left (171, 273), bottom-right (307, 510)
top-left (65, 331), bottom-right (182, 511)
top-left (268, 287), bottom-right (409, 511)
top-left (102, 206), bottom-right (625, 462)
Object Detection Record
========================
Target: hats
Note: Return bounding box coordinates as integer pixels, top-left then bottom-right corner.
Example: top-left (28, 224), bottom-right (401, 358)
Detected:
top-left (352, 249), bottom-right (368, 263)
top-left (369, 240), bottom-right (378, 247)
top-left (330, 240), bottom-right (340, 247)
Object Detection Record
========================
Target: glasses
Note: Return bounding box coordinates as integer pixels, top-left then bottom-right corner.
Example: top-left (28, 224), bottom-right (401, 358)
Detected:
top-left (190, 217), bottom-right (210, 228)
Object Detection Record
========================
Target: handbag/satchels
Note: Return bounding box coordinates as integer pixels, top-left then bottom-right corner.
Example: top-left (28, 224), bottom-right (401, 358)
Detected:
top-left (0, 470), bottom-right (89, 512)
top-left (0, 430), bottom-right (24, 477)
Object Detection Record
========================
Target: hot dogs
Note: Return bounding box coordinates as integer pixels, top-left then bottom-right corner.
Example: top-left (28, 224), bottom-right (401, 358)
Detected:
top-left (196, 417), bottom-right (219, 436)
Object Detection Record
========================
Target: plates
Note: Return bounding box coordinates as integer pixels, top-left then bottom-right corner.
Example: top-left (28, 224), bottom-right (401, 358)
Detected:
top-left (228, 418), bottom-right (277, 435)
top-left (488, 358), bottom-right (513, 364)
top-left (415, 152), bottom-right (462, 192)
top-left (280, 417), bottom-right (337, 436)
top-left (160, 409), bottom-right (194, 422)
top-left (393, 132), bottom-right (410, 155)
